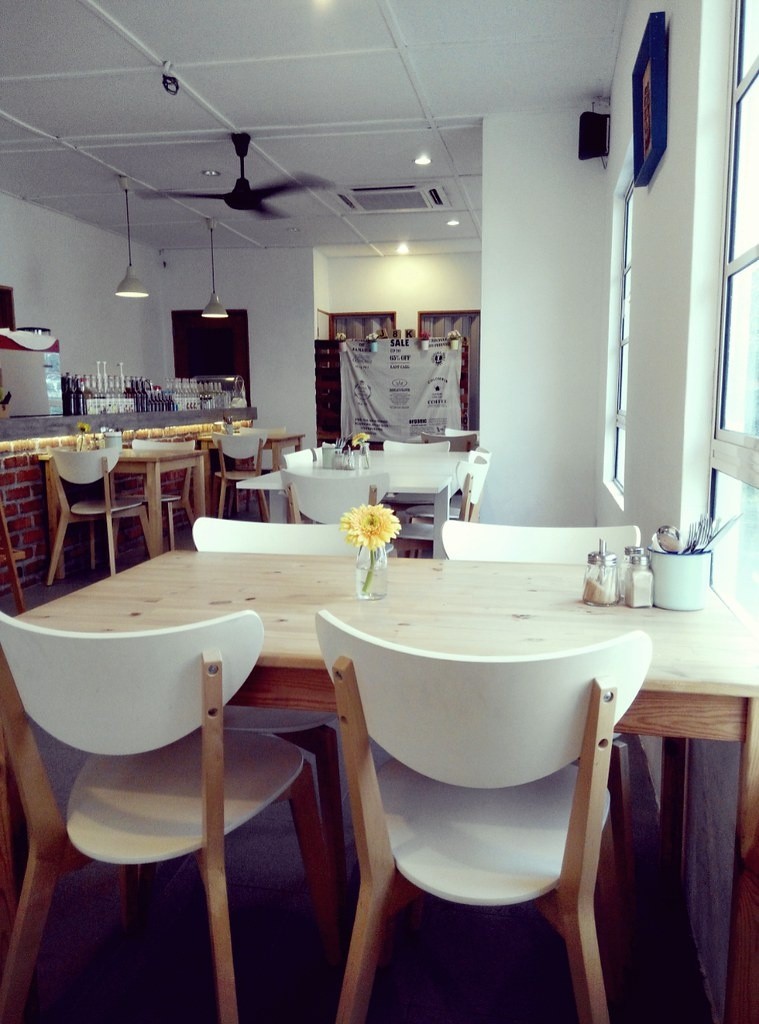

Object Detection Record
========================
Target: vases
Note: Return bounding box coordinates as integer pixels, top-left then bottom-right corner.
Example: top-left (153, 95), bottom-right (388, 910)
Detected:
top-left (421, 340), bottom-right (430, 351)
top-left (358, 442), bottom-right (371, 468)
top-left (353, 538), bottom-right (395, 601)
top-left (450, 339), bottom-right (460, 350)
top-left (339, 341), bottom-right (346, 352)
top-left (368, 341), bottom-right (378, 353)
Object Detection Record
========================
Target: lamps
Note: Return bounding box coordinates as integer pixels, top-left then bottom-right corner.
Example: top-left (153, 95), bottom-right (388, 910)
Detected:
top-left (115, 171), bottom-right (151, 298)
top-left (201, 216), bottom-right (229, 319)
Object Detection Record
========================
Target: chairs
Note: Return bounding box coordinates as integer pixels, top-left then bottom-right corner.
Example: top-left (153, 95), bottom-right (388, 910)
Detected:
top-left (405, 446), bottom-right (493, 523)
top-left (438, 519), bottom-right (643, 964)
top-left (396, 454), bottom-right (492, 556)
top-left (382, 440), bottom-right (451, 505)
top-left (313, 447), bottom-right (322, 460)
top-left (189, 517), bottom-right (353, 887)
top-left (0, 607), bottom-right (340, 1024)
top-left (275, 467), bottom-right (399, 557)
top-left (283, 448), bottom-right (314, 468)
top-left (43, 443), bottom-right (161, 586)
top-left (119, 438), bottom-right (197, 550)
top-left (239, 427), bottom-right (287, 468)
top-left (421, 432), bottom-right (477, 450)
top-left (213, 432), bottom-right (270, 521)
top-left (444, 429), bottom-right (479, 440)
top-left (307, 606), bottom-right (654, 1024)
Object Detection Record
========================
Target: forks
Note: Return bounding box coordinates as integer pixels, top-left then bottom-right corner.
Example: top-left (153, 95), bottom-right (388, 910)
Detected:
top-left (679, 514), bottom-right (721, 554)
top-left (334, 437), bottom-right (350, 448)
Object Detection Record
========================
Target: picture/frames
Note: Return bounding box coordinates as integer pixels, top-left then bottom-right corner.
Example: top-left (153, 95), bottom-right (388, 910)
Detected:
top-left (631, 11), bottom-right (666, 187)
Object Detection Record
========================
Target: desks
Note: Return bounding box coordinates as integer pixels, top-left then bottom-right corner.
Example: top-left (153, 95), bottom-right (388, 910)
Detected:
top-left (37, 447), bottom-right (212, 580)
top-left (194, 433), bottom-right (306, 517)
top-left (233, 448), bottom-right (481, 558)
top-left (0, 536), bottom-right (759, 1024)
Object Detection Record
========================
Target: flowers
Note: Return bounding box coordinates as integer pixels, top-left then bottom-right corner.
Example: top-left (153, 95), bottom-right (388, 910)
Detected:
top-left (447, 329), bottom-right (463, 340)
top-left (339, 498), bottom-right (404, 593)
top-left (419, 330), bottom-right (430, 339)
top-left (334, 332), bottom-right (347, 342)
top-left (365, 333), bottom-right (379, 342)
top-left (352, 432), bottom-right (374, 457)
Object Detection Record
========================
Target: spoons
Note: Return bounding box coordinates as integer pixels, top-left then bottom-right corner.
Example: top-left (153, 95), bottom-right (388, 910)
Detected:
top-left (657, 525), bottom-right (682, 554)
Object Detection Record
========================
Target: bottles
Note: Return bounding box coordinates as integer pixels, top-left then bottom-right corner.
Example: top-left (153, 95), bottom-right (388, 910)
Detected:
top-left (621, 545), bottom-right (653, 608)
top-left (582, 540), bottom-right (621, 607)
top-left (90, 437), bottom-right (105, 450)
top-left (61, 371), bottom-right (231, 416)
top-left (335, 450), bottom-right (355, 470)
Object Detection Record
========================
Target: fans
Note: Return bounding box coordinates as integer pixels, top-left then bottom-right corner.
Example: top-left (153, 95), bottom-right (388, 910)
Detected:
top-left (133, 132), bottom-right (339, 222)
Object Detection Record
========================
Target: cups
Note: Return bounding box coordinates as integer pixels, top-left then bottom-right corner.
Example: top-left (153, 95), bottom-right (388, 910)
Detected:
top-left (223, 424), bottom-right (233, 436)
top-left (322, 448), bottom-right (342, 469)
top-left (103, 431), bottom-right (122, 449)
top-left (647, 546), bottom-right (712, 611)
